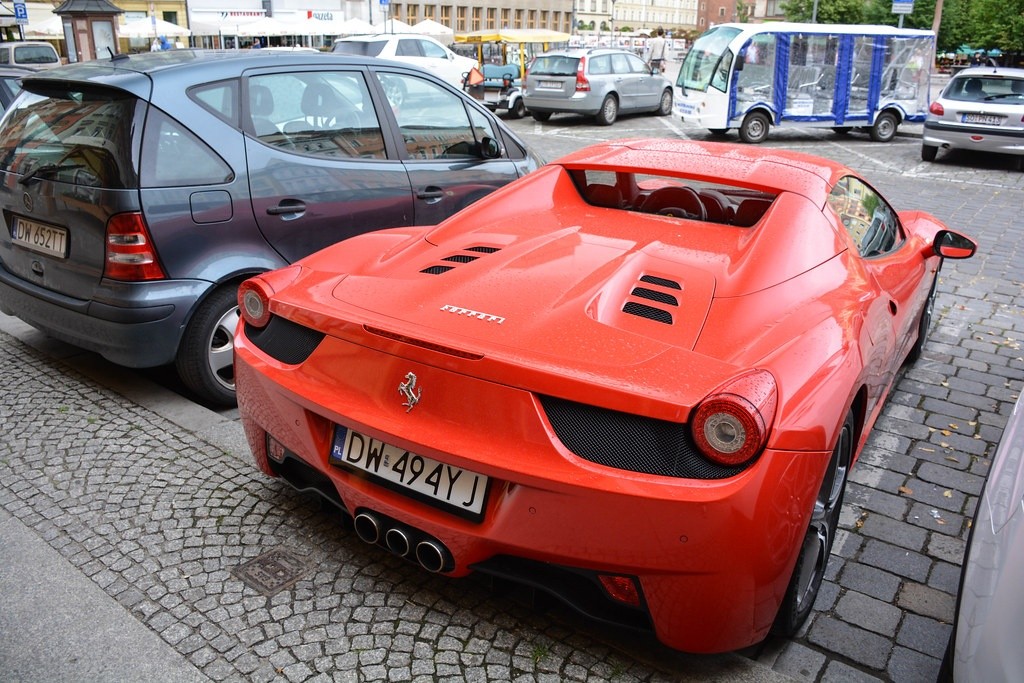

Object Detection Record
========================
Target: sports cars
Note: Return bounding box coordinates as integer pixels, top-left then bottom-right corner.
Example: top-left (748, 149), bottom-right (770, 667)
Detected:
top-left (231, 140), bottom-right (978, 654)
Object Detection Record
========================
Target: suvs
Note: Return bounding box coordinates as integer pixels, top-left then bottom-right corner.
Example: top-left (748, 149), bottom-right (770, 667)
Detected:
top-left (520, 47), bottom-right (673, 125)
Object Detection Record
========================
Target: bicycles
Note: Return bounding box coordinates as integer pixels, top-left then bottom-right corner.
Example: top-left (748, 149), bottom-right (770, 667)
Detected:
top-left (920, 68), bottom-right (929, 86)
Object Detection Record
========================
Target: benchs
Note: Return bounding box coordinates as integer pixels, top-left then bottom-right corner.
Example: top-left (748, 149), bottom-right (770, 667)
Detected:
top-left (811, 64), bottom-right (860, 100)
top-left (756, 63), bottom-right (824, 99)
top-left (479, 63), bottom-right (521, 92)
top-left (850, 64), bottom-right (895, 100)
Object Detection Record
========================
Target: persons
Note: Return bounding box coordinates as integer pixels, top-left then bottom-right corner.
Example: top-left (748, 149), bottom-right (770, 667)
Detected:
top-left (909, 48), bottom-right (923, 82)
top-left (745, 40), bottom-right (760, 64)
top-left (252, 38), bottom-right (260, 49)
top-left (159, 36), bottom-right (172, 50)
top-left (151, 39), bottom-right (162, 53)
top-left (969, 52), bottom-right (982, 67)
top-left (647, 28), bottom-right (669, 73)
top-left (175, 36), bottom-right (184, 49)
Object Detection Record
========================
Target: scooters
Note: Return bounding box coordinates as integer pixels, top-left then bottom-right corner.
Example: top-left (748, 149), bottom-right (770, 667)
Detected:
top-left (454, 29), bottom-right (572, 118)
top-left (671, 21), bottom-right (936, 143)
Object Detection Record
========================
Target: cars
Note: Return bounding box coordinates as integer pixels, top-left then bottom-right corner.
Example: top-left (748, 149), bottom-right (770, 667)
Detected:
top-left (0, 63), bottom-right (40, 124)
top-left (921, 68), bottom-right (1024, 162)
top-left (329, 33), bottom-right (481, 108)
top-left (0, 48), bottom-right (548, 410)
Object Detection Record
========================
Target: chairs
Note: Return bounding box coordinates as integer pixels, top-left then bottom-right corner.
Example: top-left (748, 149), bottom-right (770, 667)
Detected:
top-left (1011, 81), bottom-right (1024, 94)
top-left (294, 82), bottom-right (341, 156)
top-left (733, 198), bottom-right (772, 226)
top-left (965, 80), bottom-right (983, 98)
top-left (584, 184), bottom-right (623, 210)
top-left (249, 85), bottom-right (295, 151)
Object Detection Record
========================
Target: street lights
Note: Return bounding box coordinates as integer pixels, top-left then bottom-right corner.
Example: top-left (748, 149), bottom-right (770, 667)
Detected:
top-left (611, 0), bottom-right (617, 45)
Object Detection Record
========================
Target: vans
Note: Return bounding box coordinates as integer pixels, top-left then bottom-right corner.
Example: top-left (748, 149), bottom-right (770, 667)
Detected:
top-left (0, 41), bottom-right (62, 69)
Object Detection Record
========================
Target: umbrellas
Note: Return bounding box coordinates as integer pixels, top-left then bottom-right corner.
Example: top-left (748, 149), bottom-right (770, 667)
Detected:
top-left (376, 19), bottom-right (418, 33)
top-left (413, 19), bottom-right (454, 37)
top-left (340, 18), bottom-right (383, 35)
top-left (297, 17), bottom-right (343, 48)
top-left (25, 16), bottom-right (66, 58)
top-left (117, 16), bottom-right (192, 52)
top-left (236, 16), bottom-right (295, 47)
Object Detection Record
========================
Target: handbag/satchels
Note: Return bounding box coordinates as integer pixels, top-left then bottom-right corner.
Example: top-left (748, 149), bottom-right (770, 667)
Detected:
top-left (659, 40), bottom-right (667, 73)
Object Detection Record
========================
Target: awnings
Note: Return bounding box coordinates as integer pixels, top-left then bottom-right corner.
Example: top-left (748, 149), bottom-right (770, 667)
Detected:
top-left (956, 47), bottom-right (1002, 57)
